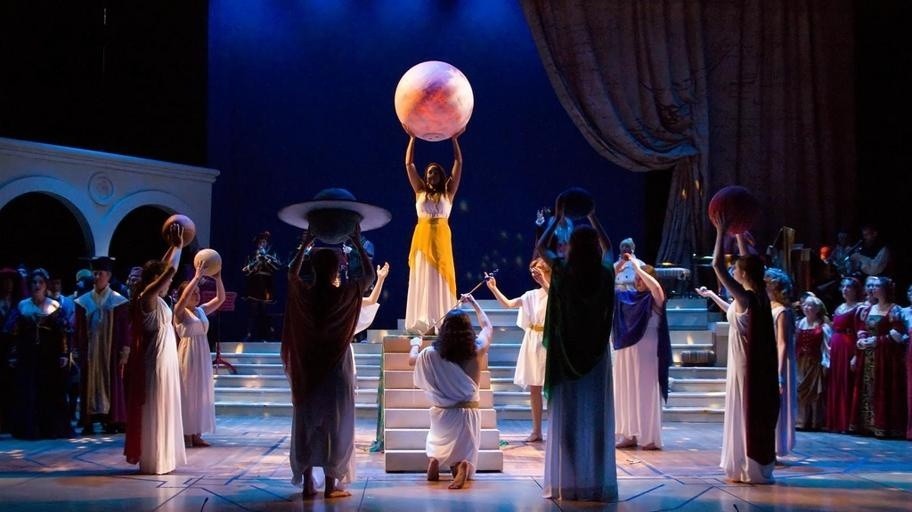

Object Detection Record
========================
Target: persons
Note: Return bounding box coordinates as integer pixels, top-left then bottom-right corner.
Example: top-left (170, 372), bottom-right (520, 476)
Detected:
top-left (611, 235), bottom-right (647, 295)
top-left (290, 261), bottom-right (390, 370)
top-left (484, 254), bottom-right (551, 443)
top-left (282, 223), bottom-right (375, 499)
top-left (534, 204), bottom-right (571, 258)
top-left (402, 121), bottom-right (463, 364)
top-left (241, 229), bottom-right (279, 337)
top-left (0, 220), bottom-right (226, 476)
top-left (411, 293), bottom-right (494, 489)
top-left (533, 196), bottom-right (619, 502)
top-left (613, 256), bottom-right (668, 450)
